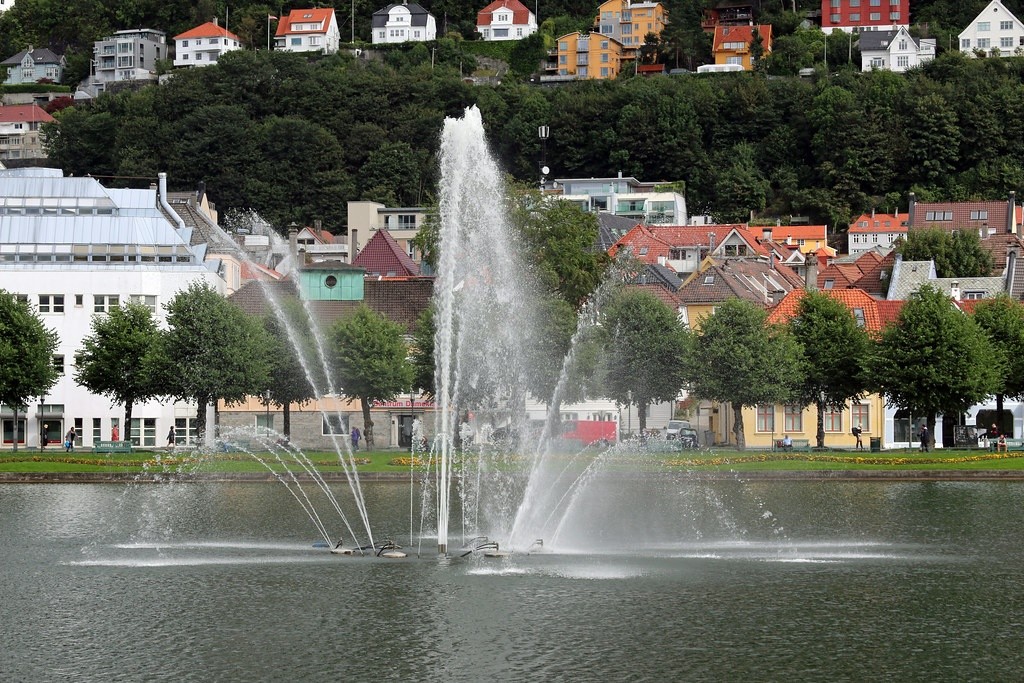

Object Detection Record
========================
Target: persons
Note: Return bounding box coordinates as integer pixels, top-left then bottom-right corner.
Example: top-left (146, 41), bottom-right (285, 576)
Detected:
top-left (783, 435), bottom-right (793, 452)
top-left (352, 426), bottom-right (361, 450)
top-left (40, 425), bottom-right (48, 449)
top-left (991, 423), bottom-right (1000, 437)
top-left (165, 426), bottom-right (175, 450)
top-left (997, 434), bottom-right (1007, 452)
top-left (917, 424), bottom-right (930, 453)
top-left (112, 424), bottom-right (119, 441)
top-left (65, 427), bottom-right (78, 452)
top-left (856, 423), bottom-right (863, 449)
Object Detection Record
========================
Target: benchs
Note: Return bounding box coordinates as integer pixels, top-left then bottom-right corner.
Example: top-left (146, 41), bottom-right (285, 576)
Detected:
top-left (92, 440), bottom-right (131, 454)
top-left (773, 439), bottom-right (812, 453)
top-left (989, 438), bottom-right (1024, 453)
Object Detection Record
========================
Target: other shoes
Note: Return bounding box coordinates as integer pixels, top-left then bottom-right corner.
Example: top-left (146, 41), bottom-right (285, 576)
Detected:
top-left (356, 448), bottom-right (358, 451)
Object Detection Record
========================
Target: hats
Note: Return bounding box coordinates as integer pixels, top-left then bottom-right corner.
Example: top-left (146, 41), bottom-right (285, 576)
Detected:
top-left (44, 424), bottom-right (49, 426)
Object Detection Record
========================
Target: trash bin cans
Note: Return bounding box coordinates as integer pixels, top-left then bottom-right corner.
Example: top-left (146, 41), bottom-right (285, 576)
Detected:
top-left (870, 436), bottom-right (881, 452)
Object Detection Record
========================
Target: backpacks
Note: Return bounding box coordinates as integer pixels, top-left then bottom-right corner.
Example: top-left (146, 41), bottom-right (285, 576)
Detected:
top-left (356, 428), bottom-right (361, 440)
top-left (851, 426), bottom-right (861, 436)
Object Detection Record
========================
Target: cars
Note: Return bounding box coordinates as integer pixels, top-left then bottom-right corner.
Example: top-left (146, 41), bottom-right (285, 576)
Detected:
top-left (459, 425), bottom-right (475, 444)
top-left (663, 419), bottom-right (690, 440)
top-left (491, 419), bottom-right (546, 446)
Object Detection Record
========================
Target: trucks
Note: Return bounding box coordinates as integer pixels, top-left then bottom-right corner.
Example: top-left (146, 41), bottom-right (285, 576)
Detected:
top-left (559, 419), bottom-right (616, 450)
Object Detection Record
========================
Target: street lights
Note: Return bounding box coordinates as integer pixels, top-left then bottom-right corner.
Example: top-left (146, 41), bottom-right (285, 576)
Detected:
top-left (263, 389), bottom-right (275, 445)
top-left (40, 394), bottom-right (46, 452)
top-left (410, 390), bottom-right (415, 422)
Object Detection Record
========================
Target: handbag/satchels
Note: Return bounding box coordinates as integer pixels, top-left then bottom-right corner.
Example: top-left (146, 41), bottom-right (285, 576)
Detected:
top-left (44, 434), bottom-right (49, 439)
top-left (994, 442), bottom-right (998, 447)
top-left (65, 440), bottom-right (69, 448)
